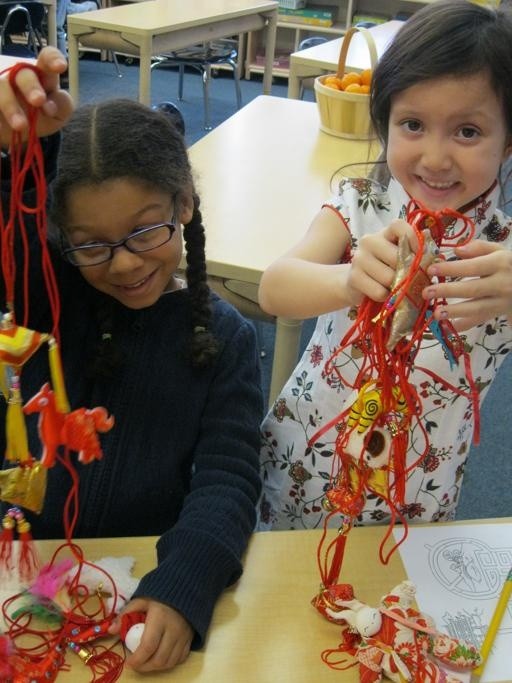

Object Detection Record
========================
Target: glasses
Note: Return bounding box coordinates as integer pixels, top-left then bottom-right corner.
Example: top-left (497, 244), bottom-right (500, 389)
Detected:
top-left (59, 193), bottom-right (180, 267)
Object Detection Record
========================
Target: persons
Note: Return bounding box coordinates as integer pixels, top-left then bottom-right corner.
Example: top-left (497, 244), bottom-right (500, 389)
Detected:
top-left (251, 0), bottom-right (511, 533)
top-left (0, 44), bottom-right (264, 676)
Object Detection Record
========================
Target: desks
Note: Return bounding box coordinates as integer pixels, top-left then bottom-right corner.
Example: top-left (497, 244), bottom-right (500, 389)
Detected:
top-left (66, 0), bottom-right (279, 108)
top-left (183, 93), bottom-right (387, 411)
top-left (1, 514), bottom-right (512, 682)
top-left (48, 0), bottom-right (437, 98)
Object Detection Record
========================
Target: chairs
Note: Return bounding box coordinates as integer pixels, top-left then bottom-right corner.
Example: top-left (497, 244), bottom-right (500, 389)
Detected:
top-left (149, 48), bottom-right (245, 129)
top-left (0, 2), bottom-right (46, 54)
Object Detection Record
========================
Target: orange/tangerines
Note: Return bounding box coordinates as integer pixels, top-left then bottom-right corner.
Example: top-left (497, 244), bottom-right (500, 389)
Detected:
top-left (325, 69), bottom-right (374, 94)
